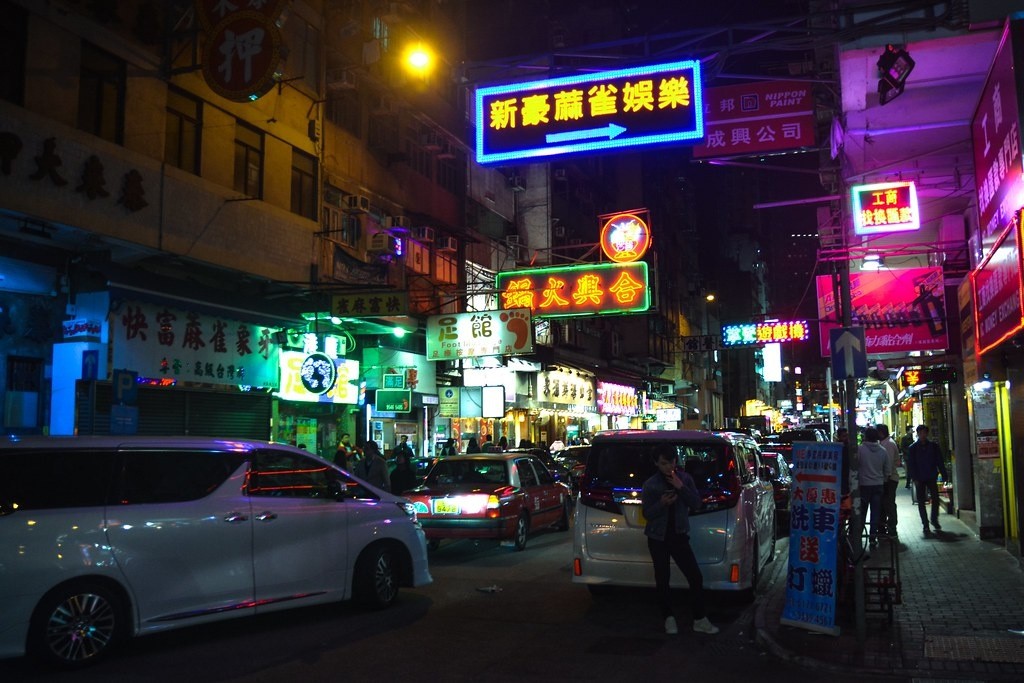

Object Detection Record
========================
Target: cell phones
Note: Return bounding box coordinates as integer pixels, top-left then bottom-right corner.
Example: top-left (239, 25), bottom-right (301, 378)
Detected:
top-left (666, 490), bottom-right (674, 497)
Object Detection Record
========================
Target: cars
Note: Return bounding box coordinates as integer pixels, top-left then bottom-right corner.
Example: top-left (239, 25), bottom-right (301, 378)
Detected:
top-left (738, 414), bottom-right (839, 536)
top-left (401, 446), bottom-right (575, 552)
top-left (475, 444), bottom-right (593, 508)
top-left (0, 432), bottom-right (434, 672)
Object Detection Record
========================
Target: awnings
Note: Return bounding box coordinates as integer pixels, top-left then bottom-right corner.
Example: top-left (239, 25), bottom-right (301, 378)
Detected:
top-left (556, 359), bottom-right (643, 389)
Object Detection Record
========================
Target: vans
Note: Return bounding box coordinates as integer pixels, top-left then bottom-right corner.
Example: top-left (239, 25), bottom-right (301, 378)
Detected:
top-left (570, 428), bottom-right (784, 605)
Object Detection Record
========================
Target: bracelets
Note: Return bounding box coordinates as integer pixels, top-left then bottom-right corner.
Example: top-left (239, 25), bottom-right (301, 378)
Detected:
top-left (679, 484), bottom-right (686, 491)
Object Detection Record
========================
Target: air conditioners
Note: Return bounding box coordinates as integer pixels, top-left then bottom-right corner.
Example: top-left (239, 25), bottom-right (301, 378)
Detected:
top-left (416, 226), bottom-right (434, 242)
top-left (506, 235), bottom-right (522, 259)
top-left (367, 234), bottom-right (401, 256)
top-left (606, 331), bottom-right (618, 356)
top-left (939, 214), bottom-right (966, 266)
top-left (508, 176), bottom-right (526, 191)
top-left (424, 134), bottom-right (456, 160)
top-left (285, 327), bottom-right (303, 349)
top-left (322, 335), bottom-right (346, 355)
top-left (660, 384), bottom-right (674, 395)
top-left (342, 194), bottom-right (369, 213)
top-left (561, 325), bottom-right (586, 348)
top-left (385, 216), bottom-right (411, 230)
top-left (556, 169), bottom-right (567, 180)
top-left (326, 70), bottom-right (354, 90)
top-left (556, 226), bottom-right (565, 237)
top-left (437, 236), bottom-right (457, 252)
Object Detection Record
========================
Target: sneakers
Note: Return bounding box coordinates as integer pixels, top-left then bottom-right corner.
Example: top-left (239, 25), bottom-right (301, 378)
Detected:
top-left (694, 617), bottom-right (718, 633)
top-left (664, 616), bottom-right (678, 634)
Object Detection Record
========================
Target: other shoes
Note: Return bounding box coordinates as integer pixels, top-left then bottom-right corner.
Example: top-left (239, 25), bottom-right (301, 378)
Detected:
top-left (870, 540), bottom-right (878, 546)
top-left (880, 532), bottom-right (898, 540)
top-left (924, 526), bottom-right (933, 537)
top-left (931, 521), bottom-right (941, 529)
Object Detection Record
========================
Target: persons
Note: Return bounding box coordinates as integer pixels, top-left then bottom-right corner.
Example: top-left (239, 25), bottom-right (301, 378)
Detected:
top-left (441, 438), bottom-right (455, 457)
top-left (901, 425), bottom-right (948, 535)
top-left (332, 433), bottom-right (391, 493)
top-left (549, 437), bottom-right (564, 451)
top-left (298, 443), bottom-right (307, 451)
top-left (642, 445), bottom-right (720, 635)
top-left (539, 442), bottom-right (551, 453)
top-left (850, 425), bottom-right (899, 547)
top-left (482, 435), bottom-right (496, 453)
top-left (466, 437), bottom-right (480, 455)
top-left (571, 437), bottom-right (589, 446)
top-left (498, 435), bottom-right (509, 452)
top-left (390, 436), bottom-right (415, 458)
top-left (834, 428), bottom-right (854, 495)
top-left (388, 450), bottom-right (418, 497)
top-left (519, 439), bottom-right (535, 448)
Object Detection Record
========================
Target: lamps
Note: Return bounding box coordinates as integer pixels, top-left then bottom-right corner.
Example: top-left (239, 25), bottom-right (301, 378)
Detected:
top-left (877, 44), bottom-right (915, 106)
